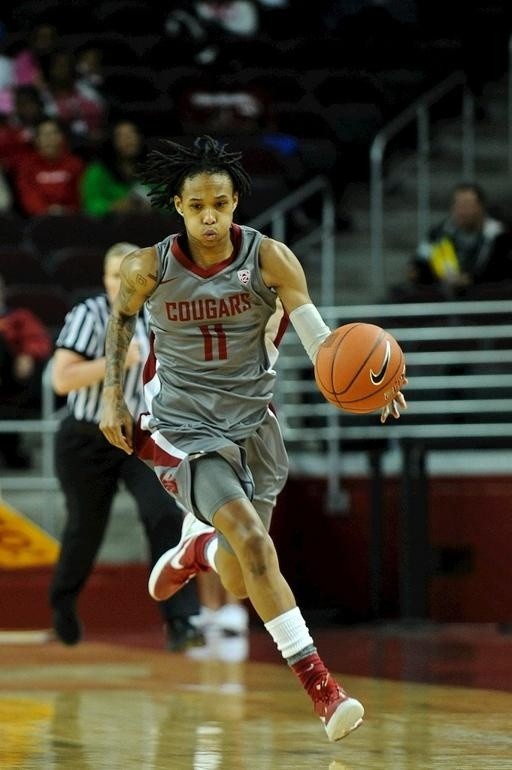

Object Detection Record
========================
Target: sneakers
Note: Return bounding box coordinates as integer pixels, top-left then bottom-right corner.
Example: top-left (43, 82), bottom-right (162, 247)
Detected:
top-left (312, 674), bottom-right (365, 742)
top-left (148, 512), bottom-right (216, 603)
top-left (51, 602), bottom-right (82, 645)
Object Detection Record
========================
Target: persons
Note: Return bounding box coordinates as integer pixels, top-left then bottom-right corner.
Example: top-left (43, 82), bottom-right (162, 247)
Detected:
top-left (0, 280), bottom-right (53, 470)
top-left (96, 135), bottom-right (410, 742)
top-left (395, 175), bottom-right (512, 301)
top-left (49, 240), bottom-right (201, 654)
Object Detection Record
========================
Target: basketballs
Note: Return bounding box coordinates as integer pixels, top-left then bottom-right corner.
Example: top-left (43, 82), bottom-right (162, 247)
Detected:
top-left (312, 323), bottom-right (407, 415)
